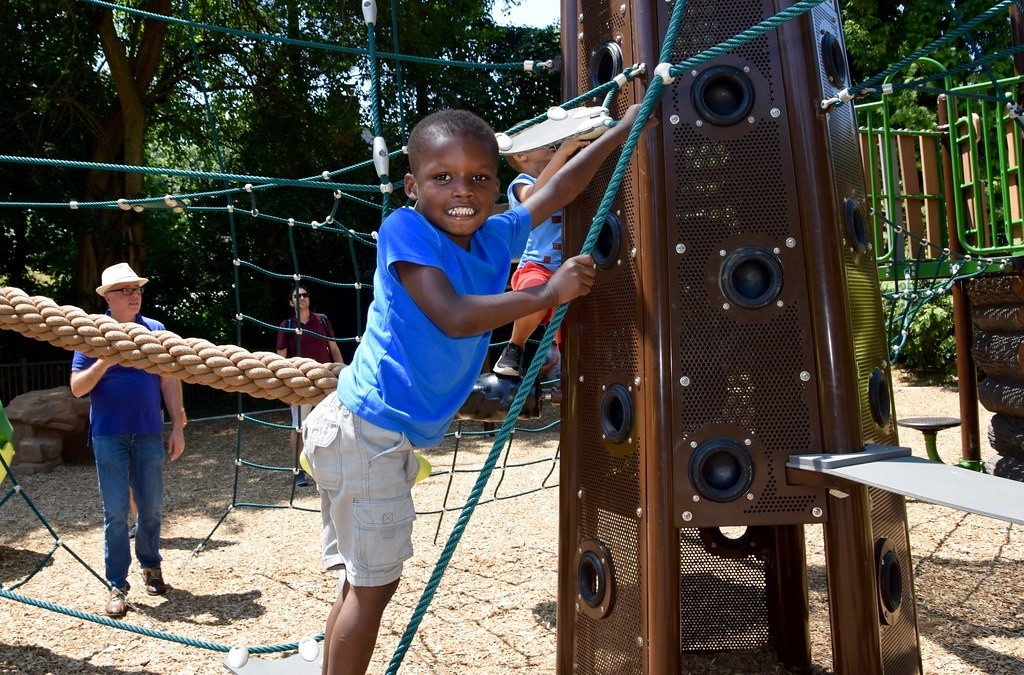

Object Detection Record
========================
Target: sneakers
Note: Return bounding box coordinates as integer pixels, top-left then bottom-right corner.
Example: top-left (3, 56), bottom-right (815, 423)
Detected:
top-left (128, 524), bottom-right (139, 538)
top-left (493, 341), bottom-right (523, 376)
top-left (295, 472), bottom-right (307, 486)
top-left (525, 321), bottom-right (551, 367)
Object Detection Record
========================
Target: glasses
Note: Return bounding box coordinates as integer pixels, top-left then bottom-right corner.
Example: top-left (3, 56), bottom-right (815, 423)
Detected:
top-left (293, 293), bottom-right (310, 299)
top-left (106, 287), bottom-right (145, 295)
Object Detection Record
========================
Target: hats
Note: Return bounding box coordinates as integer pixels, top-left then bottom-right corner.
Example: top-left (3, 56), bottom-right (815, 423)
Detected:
top-left (95, 262), bottom-right (149, 297)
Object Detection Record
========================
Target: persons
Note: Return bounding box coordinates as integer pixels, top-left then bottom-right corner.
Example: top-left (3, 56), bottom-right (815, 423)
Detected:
top-left (69, 262), bottom-right (186, 617)
top-left (276, 287), bottom-right (345, 485)
top-left (295, 104), bottom-right (659, 675)
top-left (492, 116), bottom-right (591, 376)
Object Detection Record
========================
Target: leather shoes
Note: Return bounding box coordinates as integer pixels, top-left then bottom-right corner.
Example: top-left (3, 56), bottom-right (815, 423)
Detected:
top-left (106, 586), bottom-right (128, 615)
top-left (143, 566), bottom-right (166, 595)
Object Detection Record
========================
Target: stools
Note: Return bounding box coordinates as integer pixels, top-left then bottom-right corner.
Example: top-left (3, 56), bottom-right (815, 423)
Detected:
top-left (897, 417), bottom-right (962, 465)
